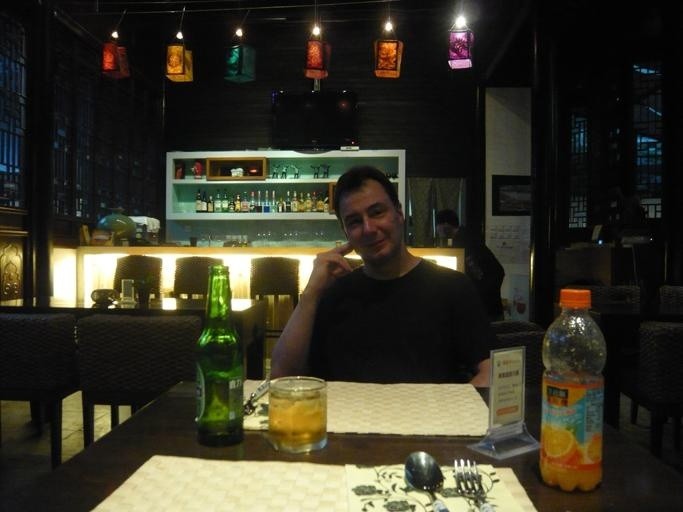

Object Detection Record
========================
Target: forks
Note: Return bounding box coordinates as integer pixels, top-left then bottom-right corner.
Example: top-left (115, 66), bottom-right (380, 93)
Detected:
top-left (454, 459), bottom-right (496, 512)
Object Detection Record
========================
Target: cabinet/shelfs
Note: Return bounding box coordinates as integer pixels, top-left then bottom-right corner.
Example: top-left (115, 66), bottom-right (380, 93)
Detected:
top-left (76, 246), bottom-right (465, 307)
top-left (166, 148), bottom-right (405, 247)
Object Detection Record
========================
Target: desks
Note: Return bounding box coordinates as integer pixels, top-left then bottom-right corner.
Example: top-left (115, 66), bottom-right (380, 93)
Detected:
top-left (0, 298), bottom-right (268, 381)
top-left (0, 379), bottom-right (683, 512)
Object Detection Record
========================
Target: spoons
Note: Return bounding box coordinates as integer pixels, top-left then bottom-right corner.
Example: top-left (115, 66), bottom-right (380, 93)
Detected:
top-left (405, 451), bottom-right (450, 512)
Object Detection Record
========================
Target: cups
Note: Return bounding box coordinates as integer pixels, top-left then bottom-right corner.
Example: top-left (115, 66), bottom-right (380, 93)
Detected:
top-left (122, 279), bottom-right (134, 303)
top-left (268, 376), bottom-right (328, 452)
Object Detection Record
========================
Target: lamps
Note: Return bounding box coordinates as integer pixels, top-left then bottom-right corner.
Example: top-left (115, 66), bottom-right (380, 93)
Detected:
top-left (103, 12), bottom-right (130, 79)
top-left (373, 1), bottom-right (404, 78)
top-left (166, 11), bottom-right (193, 83)
top-left (447, 3), bottom-right (475, 70)
top-left (223, 12), bottom-right (258, 84)
top-left (303, 5), bottom-right (331, 78)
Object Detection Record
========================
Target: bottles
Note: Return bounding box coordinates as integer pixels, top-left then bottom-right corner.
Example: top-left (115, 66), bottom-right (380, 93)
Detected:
top-left (207, 194), bottom-right (213, 212)
top-left (201, 192), bottom-right (207, 212)
top-left (540, 289), bottom-right (606, 492)
top-left (299, 192), bottom-right (306, 211)
top-left (235, 195), bottom-right (240, 212)
top-left (229, 200), bottom-right (235, 213)
top-left (277, 197), bottom-right (284, 212)
top-left (249, 192), bottom-right (256, 213)
top-left (291, 191), bottom-right (299, 212)
top-left (285, 190), bottom-right (291, 212)
top-left (256, 190), bottom-right (263, 212)
top-left (264, 190), bottom-right (270, 213)
top-left (241, 192), bottom-right (250, 213)
top-left (271, 190), bottom-right (276, 212)
top-left (312, 192), bottom-right (317, 212)
top-left (317, 190), bottom-right (324, 212)
top-left (324, 202), bottom-right (329, 212)
top-left (196, 189), bottom-right (201, 213)
top-left (305, 193), bottom-right (312, 211)
top-left (193, 264), bottom-right (245, 446)
top-left (221, 193), bottom-right (228, 212)
top-left (214, 192), bottom-right (221, 213)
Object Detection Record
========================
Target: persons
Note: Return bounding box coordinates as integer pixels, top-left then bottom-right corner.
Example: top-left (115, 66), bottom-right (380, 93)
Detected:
top-left (270, 166), bottom-right (496, 387)
top-left (434, 208), bottom-right (506, 321)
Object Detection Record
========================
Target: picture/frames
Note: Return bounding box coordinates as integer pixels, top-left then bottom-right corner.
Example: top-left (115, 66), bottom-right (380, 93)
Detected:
top-left (491, 175), bottom-right (531, 216)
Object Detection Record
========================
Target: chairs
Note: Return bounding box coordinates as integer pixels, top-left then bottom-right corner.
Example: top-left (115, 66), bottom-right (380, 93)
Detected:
top-left (568, 285), bottom-right (641, 324)
top-left (76, 314), bottom-right (203, 448)
top-left (113, 255), bottom-right (162, 299)
top-left (174, 257), bottom-right (223, 299)
top-left (0, 312), bottom-right (78, 471)
top-left (606, 319), bottom-right (683, 458)
top-left (491, 320), bottom-right (547, 385)
top-left (250, 258), bottom-right (299, 309)
top-left (659, 285), bottom-right (683, 318)
